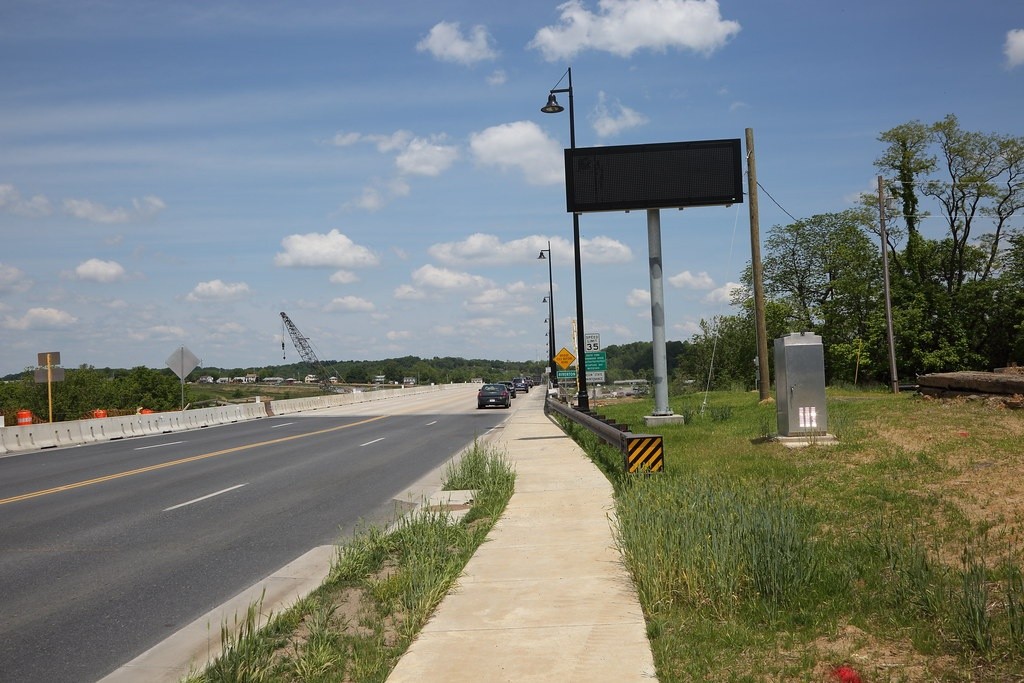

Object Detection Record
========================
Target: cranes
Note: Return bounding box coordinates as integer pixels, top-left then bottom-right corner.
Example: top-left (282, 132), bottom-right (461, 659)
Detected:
top-left (279, 310), bottom-right (361, 395)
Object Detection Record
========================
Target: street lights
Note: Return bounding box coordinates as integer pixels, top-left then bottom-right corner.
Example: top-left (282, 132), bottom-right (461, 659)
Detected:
top-left (540, 66), bottom-right (592, 413)
top-left (540, 297), bottom-right (554, 384)
top-left (537, 240), bottom-right (561, 394)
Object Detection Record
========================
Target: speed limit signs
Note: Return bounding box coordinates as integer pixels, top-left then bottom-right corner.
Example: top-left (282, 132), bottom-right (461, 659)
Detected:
top-left (584, 333), bottom-right (600, 352)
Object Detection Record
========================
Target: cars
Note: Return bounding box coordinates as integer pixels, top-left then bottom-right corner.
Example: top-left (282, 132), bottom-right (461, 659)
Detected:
top-left (477, 384), bottom-right (512, 408)
top-left (496, 381), bottom-right (516, 399)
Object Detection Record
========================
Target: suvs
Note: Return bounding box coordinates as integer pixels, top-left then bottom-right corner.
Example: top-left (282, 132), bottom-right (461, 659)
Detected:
top-left (512, 377), bottom-right (529, 393)
top-left (525, 376), bottom-right (534, 388)
